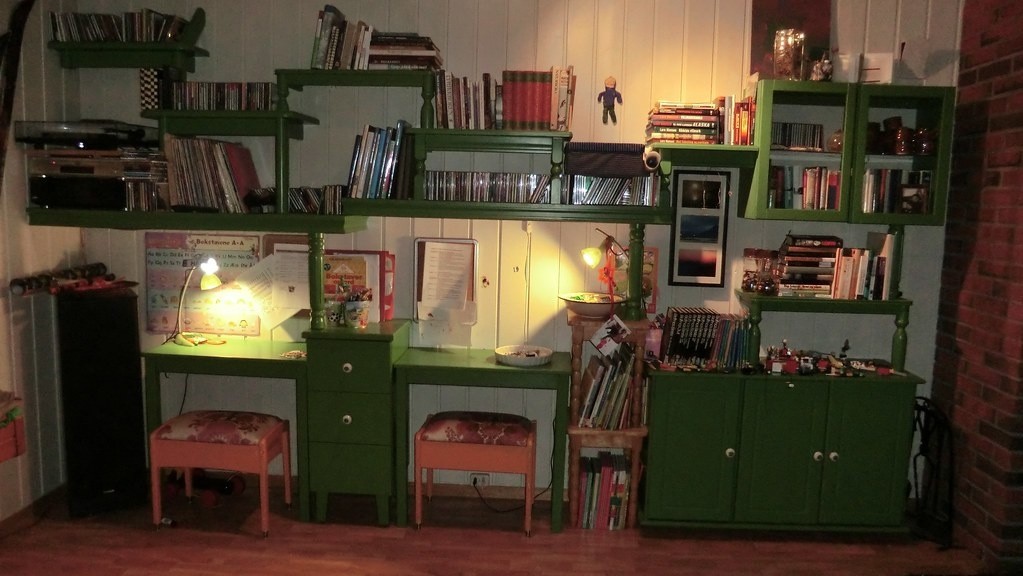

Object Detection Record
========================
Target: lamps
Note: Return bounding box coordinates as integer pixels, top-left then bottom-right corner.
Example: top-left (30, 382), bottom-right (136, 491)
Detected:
top-left (581, 227), bottom-right (629, 270)
top-left (175, 252), bottom-right (221, 346)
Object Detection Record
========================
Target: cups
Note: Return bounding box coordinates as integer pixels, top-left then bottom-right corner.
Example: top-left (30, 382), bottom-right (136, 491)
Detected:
top-left (830, 51), bottom-right (894, 85)
top-left (323, 299), bottom-right (371, 329)
top-left (773, 28), bottom-right (806, 82)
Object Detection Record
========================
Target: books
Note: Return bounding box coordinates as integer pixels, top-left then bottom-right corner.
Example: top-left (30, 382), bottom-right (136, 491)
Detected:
top-left (49, 8), bottom-right (188, 42)
top-left (311, 4), bottom-right (444, 71)
top-left (173, 81), bottom-right (278, 111)
top-left (577, 343), bottom-right (648, 428)
top-left (645, 72), bottom-right (760, 146)
top-left (777, 232), bottom-right (895, 301)
top-left (659, 306), bottom-right (749, 369)
top-left (768, 159), bottom-right (932, 214)
top-left (122, 132), bottom-right (275, 214)
top-left (424, 170), bottom-right (651, 206)
top-left (435, 66), bottom-right (574, 131)
top-left (576, 451), bottom-right (643, 530)
top-left (288, 120), bottom-right (412, 214)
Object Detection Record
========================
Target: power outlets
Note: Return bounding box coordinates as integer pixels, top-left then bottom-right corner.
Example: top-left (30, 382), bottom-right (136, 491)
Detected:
top-left (471, 473), bottom-right (489, 487)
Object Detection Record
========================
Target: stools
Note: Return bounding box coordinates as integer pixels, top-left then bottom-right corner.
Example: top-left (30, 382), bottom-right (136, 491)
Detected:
top-left (414, 411), bottom-right (536, 536)
top-left (149, 410), bottom-right (290, 541)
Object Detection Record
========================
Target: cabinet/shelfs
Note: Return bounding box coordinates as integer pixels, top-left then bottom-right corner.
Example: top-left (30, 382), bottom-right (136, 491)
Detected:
top-left (13, 37), bottom-right (958, 537)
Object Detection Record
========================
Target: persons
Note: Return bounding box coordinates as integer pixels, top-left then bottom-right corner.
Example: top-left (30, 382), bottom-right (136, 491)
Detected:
top-left (598, 77), bottom-right (622, 123)
top-left (598, 324), bottom-right (626, 354)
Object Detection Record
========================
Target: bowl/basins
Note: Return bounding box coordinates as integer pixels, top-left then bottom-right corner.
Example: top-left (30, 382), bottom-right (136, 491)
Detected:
top-left (557, 292), bottom-right (628, 319)
top-left (494, 345), bottom-right (554, 367)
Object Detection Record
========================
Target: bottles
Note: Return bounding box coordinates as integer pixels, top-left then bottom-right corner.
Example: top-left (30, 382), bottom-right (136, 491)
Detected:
top-left (895, 125), bottom-right (937, 156)
top-left (741, 247), bottom-right (779, 296)
top-left (827, 129), bottom-right (844, 153)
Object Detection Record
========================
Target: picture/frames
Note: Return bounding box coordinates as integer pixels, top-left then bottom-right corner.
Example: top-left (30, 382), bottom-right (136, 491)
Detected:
top-left (668, 169), bottom-right (731, 287)
